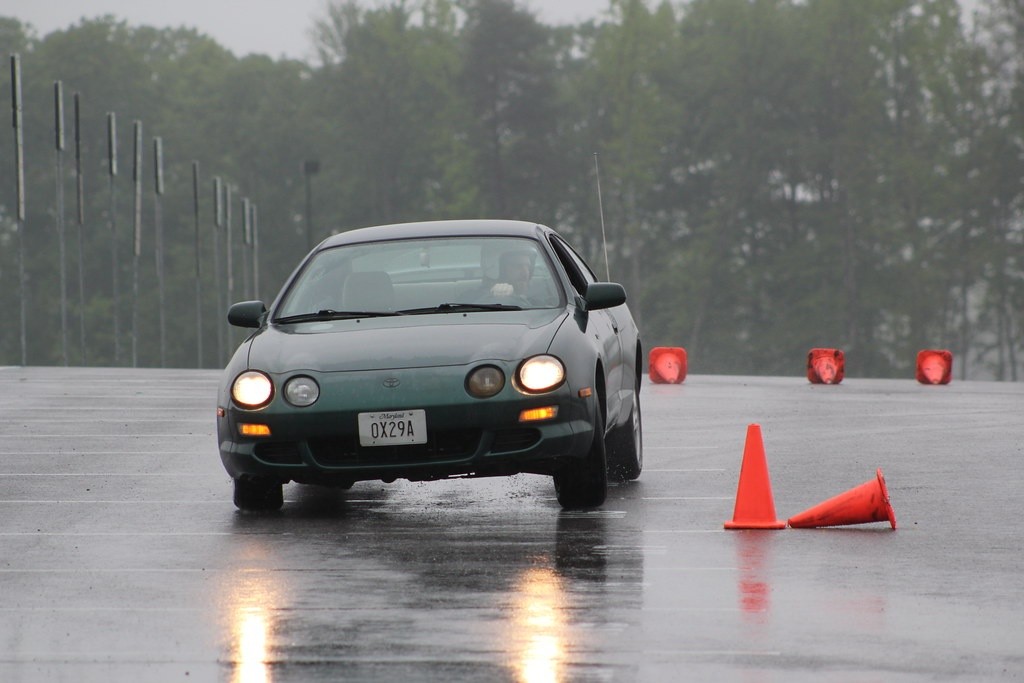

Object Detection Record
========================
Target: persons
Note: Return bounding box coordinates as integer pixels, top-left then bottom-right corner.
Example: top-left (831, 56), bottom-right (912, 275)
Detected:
top-left (475, 238), bottom-right (538, 308)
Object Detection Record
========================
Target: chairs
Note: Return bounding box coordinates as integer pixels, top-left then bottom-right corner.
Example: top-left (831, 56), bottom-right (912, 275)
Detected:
top-left (343, 272), bottom-right (394, 318)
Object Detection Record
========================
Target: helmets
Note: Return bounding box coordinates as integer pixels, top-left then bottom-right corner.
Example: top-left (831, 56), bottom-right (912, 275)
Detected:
top-left (480, 238), bottom-right (537, 280)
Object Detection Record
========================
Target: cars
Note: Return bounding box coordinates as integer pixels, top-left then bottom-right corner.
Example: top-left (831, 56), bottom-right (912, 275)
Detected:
top-left (216, 219), bottom-right (642, 510)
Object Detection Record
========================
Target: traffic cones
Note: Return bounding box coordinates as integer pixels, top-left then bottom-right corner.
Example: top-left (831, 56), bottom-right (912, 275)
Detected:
top-left (724, 423), bottom-right (786, 529)
top-left (916, 350), bottom-right (952, 385)
top-left (649, 348), bottom-right (687, 384)
top-left (807, 349), bottom-right (846, 384)
top-left (789, 470), bottom-right (895, 531)
top-left (732, 530), bottom-right (778, 612)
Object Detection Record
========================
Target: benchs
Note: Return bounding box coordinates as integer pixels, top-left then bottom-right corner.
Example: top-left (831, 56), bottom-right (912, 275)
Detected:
top-left (392, 280), bottom-right (487, 310)
